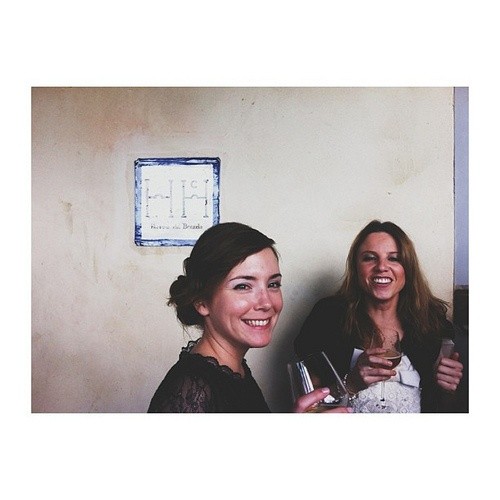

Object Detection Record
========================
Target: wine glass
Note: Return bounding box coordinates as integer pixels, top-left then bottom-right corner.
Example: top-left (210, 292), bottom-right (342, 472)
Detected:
top-left (366, 331), bottom-right (403, 413)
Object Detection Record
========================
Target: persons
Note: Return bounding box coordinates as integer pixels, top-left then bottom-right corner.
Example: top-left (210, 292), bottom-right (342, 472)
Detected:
top-left (147, 222), bottom-right (354, 413)
top-left (293, 220), bottom-right (465, 413)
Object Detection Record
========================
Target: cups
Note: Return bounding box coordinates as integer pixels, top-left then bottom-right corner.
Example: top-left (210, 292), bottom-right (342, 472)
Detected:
top-left (286, 352), bottom-right (355, 413)
